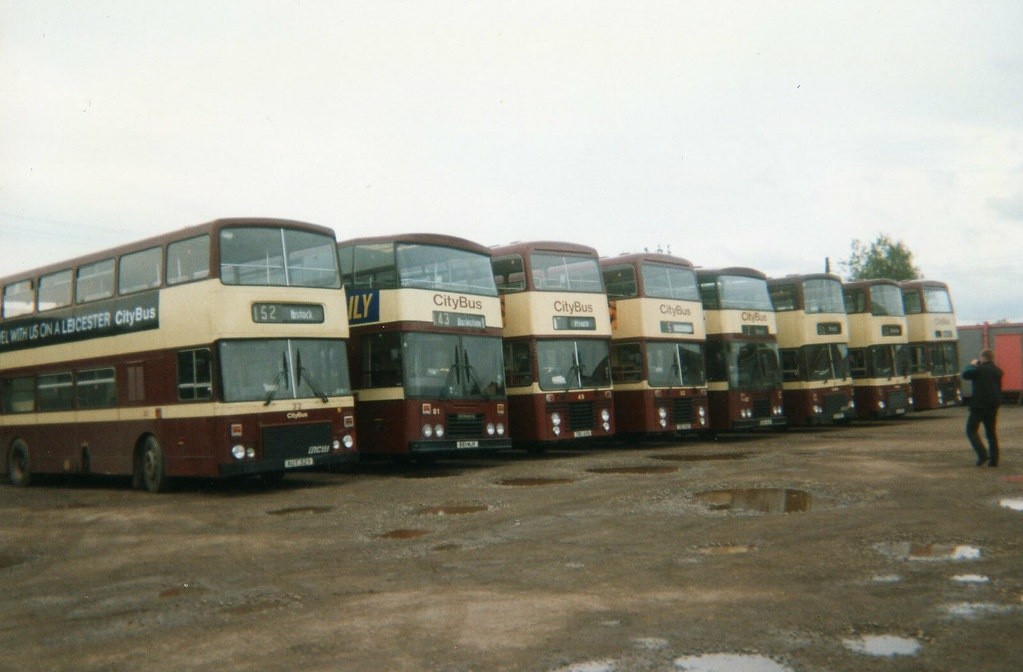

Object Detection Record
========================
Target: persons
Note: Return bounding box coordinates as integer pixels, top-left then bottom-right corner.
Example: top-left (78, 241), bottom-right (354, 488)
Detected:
top-left (961, 349), bottom-right (1005, 466)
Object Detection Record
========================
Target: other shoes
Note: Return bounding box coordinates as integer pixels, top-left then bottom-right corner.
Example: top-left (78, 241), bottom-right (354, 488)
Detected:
top-left (977, 455), bottom-right (989, 466)
top-left (988, 462), bottom-right (997, 466)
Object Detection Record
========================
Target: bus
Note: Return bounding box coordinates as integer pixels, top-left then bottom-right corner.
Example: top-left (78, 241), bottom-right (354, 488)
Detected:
top-left (699, 273), bottom-right (856, 427)
top-left (845, 282), bottom-right (965, 416)
top-left (768, 280), bottom-right (913, 423)
top-left (301, 234), bottom-right (511, 466)
top-left (608, 267), bottom-right (788, 438)
top-left (478, 240), bottom-right (617, 455)
top-left (0, 216), bottom-right (358, 497)
top-left (498, 253), bottom-right (709, 440)
